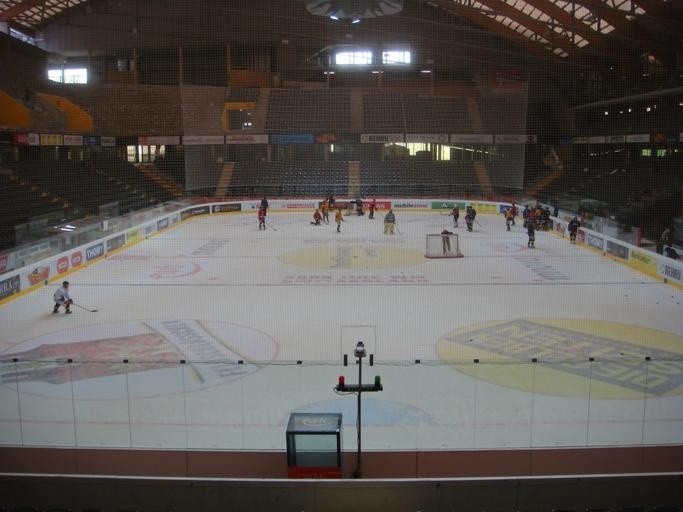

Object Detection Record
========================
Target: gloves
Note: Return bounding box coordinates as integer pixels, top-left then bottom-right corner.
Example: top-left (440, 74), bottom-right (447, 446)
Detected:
top-left (67, 298), bottom-right (72, 304)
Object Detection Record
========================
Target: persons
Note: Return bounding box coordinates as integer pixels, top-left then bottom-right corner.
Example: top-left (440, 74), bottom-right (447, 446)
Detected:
top-left (310, 195), bottom-right (379, 235)
top-left (660, 224), bottom-right (672, 241)
top-left (502, 200), bottom-right (559, 248)
top-left (261, 196), bottom-right (268, 215)
top-left (447, 206), bottom-right (459, 228)
top-left (568, 215), bottom-right (580, 243)
top-left (51, 280), bottom-right (73, 314)
top-left (258, 205), bottom-right (267, 230)
top-left (384, 208), bottom-right (395, 234)
top-left (465, 205), bottom-right (476, 232)
top-left (441, 230), bottom-right (453, 254)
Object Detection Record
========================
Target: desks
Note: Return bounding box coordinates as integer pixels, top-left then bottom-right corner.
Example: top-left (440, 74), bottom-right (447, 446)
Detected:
top-left (286, 413), bottom-right (342, 468)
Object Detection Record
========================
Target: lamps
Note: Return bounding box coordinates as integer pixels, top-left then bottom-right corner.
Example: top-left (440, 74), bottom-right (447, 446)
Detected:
top-left (305, 1), bottom-right (403, 24)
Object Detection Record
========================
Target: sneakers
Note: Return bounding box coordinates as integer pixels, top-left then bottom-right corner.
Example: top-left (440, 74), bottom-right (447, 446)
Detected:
top-left (52, 308), bottom-right (72, 313)
